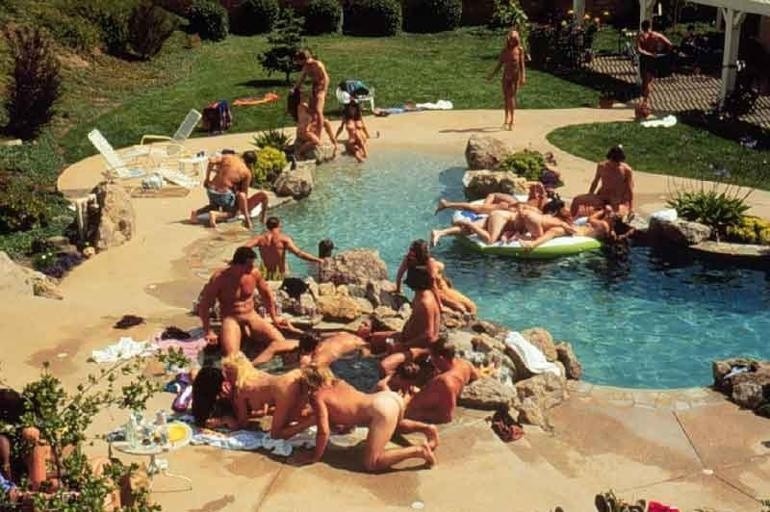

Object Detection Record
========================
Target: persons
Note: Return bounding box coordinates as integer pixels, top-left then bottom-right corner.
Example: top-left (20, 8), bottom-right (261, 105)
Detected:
top-left (486, 30), bottom-right (528, 132)
top-left (680, 24), bottom-right (703, 65)
top-left (189, 150), bottom-right (257, 228)
top-left (208, 149), bottom-right (270, 232)
top-left (241, 216), bottom-right (324, 272)
top-left (286, 86), bottom-right (341, 164)
top-left (189, 238), bottom-right (482, 476)
top-left (316, 237), bottom-right (335, 263)
top-left (334, 100), bottom-right (372, 164)
top-left (0, 386), bottom-right (153, 512)
top-left (294, 49), bottom-right (330, 141)
top-left (635, 19), bottom-right (674, 109)
top-left (427, 145), bottom-right (637, 258)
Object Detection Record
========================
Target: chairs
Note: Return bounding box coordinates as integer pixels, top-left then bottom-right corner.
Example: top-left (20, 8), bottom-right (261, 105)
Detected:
top-left (336, 79), bottom-right (375, 118)
top-left (87, 108), bottom-right (214, 197)
top-left (654, 53), bottom-right (672, 78)
top-left (682, 35), bottom-right (725, 74)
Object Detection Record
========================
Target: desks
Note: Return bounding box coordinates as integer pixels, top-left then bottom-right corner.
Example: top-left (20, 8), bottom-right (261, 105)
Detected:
top-left (111, 418), bottom-right (193, 495)
